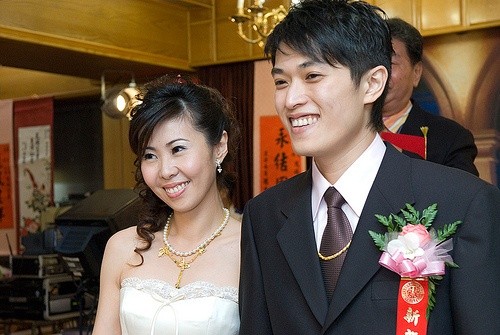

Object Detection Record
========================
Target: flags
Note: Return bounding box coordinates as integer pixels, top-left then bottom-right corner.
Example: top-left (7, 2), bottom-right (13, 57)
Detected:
top-left (380, 132), bottom-right (427, 159)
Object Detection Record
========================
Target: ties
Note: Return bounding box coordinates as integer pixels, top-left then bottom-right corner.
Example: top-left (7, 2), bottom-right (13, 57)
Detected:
top-left (319, 187), bottom-right (354, 306)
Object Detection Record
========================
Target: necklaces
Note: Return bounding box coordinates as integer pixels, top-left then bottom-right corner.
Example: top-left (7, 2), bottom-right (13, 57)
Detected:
top-left (158, 208), bottom-right (231, 288)
top-left (318, 240), bottom-right (353, 260)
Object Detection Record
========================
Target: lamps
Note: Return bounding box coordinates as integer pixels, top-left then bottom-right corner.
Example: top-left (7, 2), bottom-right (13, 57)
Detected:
top-left (98, 70), bottom-right (133, 118)
top-left (228, 0), bottom-right (288, 48)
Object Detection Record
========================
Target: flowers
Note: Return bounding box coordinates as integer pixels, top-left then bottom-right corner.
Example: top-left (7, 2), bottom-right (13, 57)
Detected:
top-left (368, 203), bottom-right (462, 320)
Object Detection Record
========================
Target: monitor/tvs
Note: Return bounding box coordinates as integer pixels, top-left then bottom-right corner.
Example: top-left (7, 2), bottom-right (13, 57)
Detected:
top-left (54, 189), bottom-right (146, 293)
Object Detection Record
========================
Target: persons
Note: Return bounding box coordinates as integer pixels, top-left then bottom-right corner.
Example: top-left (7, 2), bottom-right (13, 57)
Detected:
top-left (238, 0), bottom-right (500, 335)
top-left (92, 75), bottom-right (241, 335)
top-left (377, 18), bottom-right (480, 177)
